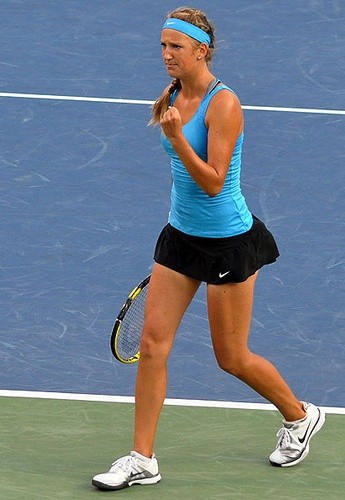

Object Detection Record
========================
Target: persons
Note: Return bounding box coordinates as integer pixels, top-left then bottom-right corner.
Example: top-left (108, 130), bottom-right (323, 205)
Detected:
top-left (92, 6), bottom-right (326, 491)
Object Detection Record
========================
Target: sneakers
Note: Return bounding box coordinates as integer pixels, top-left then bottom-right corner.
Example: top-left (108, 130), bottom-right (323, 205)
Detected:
top-left (269, 400), bottom-right (325, 467)
top-left (90, 451), bottom-right (162, 488)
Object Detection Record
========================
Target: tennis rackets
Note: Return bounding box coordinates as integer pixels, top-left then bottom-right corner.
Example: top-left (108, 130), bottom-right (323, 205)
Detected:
top-left (110, 274), bottom-right (151, 364)
